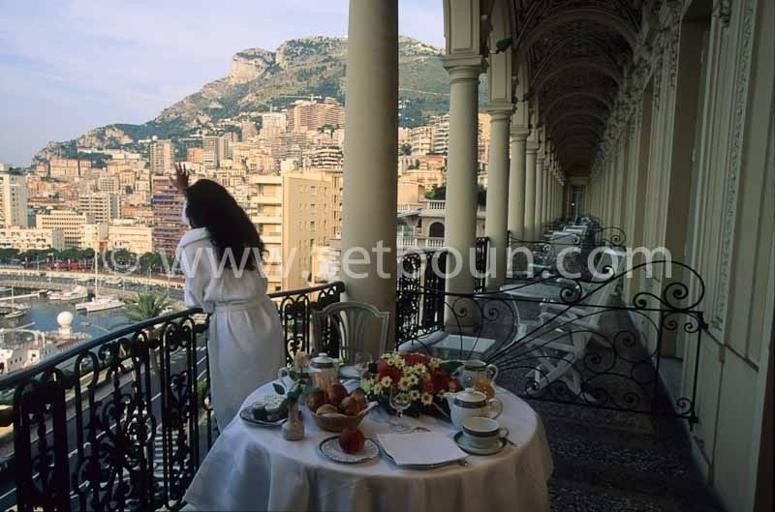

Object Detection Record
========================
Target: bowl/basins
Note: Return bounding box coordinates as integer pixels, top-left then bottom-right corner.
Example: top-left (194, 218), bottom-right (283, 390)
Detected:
top-left (305, 395), bottom-right (379, 433)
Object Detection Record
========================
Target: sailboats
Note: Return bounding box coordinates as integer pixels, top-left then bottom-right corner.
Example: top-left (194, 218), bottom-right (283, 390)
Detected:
top-left (73, 237), bottom-right (125, 312)
top-left (137, 263), bottom-right (173, 320)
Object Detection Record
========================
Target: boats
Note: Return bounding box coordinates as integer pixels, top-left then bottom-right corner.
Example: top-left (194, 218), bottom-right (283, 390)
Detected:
top-left (47, 285), bottom-right (89, 301)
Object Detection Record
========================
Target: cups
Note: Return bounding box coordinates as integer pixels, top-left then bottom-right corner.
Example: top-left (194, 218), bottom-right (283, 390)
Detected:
top-left (353, 352), bottom-right (373, 374)
top-left (462, 416), bottom-right (509, 450)
top-left (459, 359), bottom-right (499, 389)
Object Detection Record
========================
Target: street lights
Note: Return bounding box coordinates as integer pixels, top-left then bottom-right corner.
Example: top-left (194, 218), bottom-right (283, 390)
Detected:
top-left (80, 321), bottom-right (145, 439)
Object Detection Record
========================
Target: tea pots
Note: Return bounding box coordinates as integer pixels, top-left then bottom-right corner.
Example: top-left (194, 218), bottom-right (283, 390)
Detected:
top-left (309, 352), bottom-right (346, 383)
top-left (442, 387), bottom-right (504, 429)
top-left (278, 365), bottom-right (322, 406)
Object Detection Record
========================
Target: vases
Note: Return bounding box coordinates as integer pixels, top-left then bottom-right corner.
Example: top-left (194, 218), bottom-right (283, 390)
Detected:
top-left (279, 402), bottom-right (306, 442)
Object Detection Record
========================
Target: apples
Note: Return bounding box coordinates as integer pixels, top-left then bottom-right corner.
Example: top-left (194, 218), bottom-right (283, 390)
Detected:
top-left (307, 384), bottom-right (367, 416)
top-left (339, 425), bottom-right (364, 453)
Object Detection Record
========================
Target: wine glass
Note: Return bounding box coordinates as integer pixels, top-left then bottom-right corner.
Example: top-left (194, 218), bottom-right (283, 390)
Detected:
top-left (388, 383), bottom-right (413, 427)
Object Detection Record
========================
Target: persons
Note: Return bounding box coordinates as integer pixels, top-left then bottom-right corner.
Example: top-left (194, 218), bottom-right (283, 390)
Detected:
top-left (168, 160), bottom-right (288, 437)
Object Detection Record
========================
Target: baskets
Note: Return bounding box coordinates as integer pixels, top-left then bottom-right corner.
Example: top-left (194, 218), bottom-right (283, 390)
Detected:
top-left (305, 390), bottom-right (379, 431)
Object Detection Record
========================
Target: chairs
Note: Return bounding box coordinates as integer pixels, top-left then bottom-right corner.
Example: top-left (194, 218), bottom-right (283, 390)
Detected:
top-left (496, 216), bottom-right (626, 406)
top-left (309, 299), bottom-right (393, 363)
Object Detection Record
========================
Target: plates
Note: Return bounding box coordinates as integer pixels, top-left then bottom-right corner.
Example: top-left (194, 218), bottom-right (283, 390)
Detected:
top-left (239, 404), bottom-right (303, 427)
top-left (376, 431), bottom-right (470, 469)
top-left (454, 430), bottom-right (508, 456)
top-left (319, 435), bottom-right (381, 463)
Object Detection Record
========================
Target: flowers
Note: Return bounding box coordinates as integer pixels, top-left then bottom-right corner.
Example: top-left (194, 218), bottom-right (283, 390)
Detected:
top-left (358, 349), bottom-right (466, 422)
top-left (273, 351), bottom-right (322, 414)
